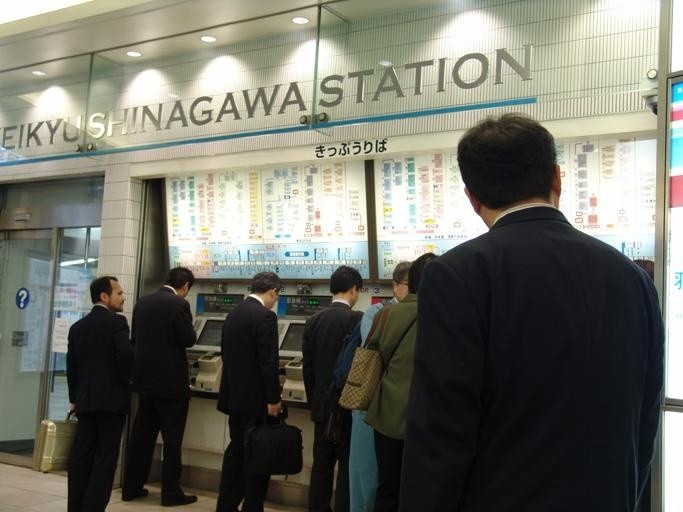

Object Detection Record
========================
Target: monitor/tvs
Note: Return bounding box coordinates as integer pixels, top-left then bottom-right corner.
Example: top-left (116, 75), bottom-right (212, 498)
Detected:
top-left (196, 320), bottom-right (224, 346)
top-left (279, 324), bottom-right (305, 351)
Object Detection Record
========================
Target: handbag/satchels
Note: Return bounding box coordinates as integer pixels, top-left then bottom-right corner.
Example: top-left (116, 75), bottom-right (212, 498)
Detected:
top-left (338, 346), bottom-right (382, 410)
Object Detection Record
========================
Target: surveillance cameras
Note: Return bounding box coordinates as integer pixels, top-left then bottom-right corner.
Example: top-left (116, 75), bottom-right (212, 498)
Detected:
top-left (639, 88), bottom-right (657, 114)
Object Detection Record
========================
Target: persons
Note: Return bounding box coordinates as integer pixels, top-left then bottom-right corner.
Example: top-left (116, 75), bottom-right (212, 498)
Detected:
top-left (347, 260), bottom-right (413, 511)
top-left (215, 270), bottom-right (284, 511)
top-left (369, 253), bottom-right (449, 512)
top-left (301, 265), bottom-right (365, 511)
top-left (121, 267), bottom-right (197, 506)
top-left (394, 110), bottom-right (666, 510)
top-left (631, 259), bottom-right (655, 283)
top-left (65, 275), bottom-right (136, 511)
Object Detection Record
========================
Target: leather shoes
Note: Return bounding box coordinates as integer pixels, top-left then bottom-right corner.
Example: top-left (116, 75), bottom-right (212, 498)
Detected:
top-left (162, 491), bottom-right (197, 505)
top-left (122, 488), bottom-right (148, 500)
